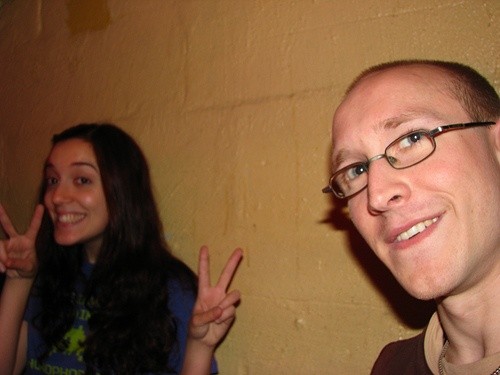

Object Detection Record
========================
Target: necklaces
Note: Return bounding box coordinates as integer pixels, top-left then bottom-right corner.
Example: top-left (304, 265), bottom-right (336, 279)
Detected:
top-left (438, 338), bottom-right (500, 375)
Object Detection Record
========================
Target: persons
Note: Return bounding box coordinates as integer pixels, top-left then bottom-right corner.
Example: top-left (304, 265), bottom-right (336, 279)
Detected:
top-left (322, 59), bottom-right (500, 375)
top-left (0, 123), bottom-right (243, 375)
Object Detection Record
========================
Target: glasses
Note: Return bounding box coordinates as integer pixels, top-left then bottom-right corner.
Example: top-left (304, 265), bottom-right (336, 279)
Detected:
top-left (322, 121), bottom-right (496, 200)
